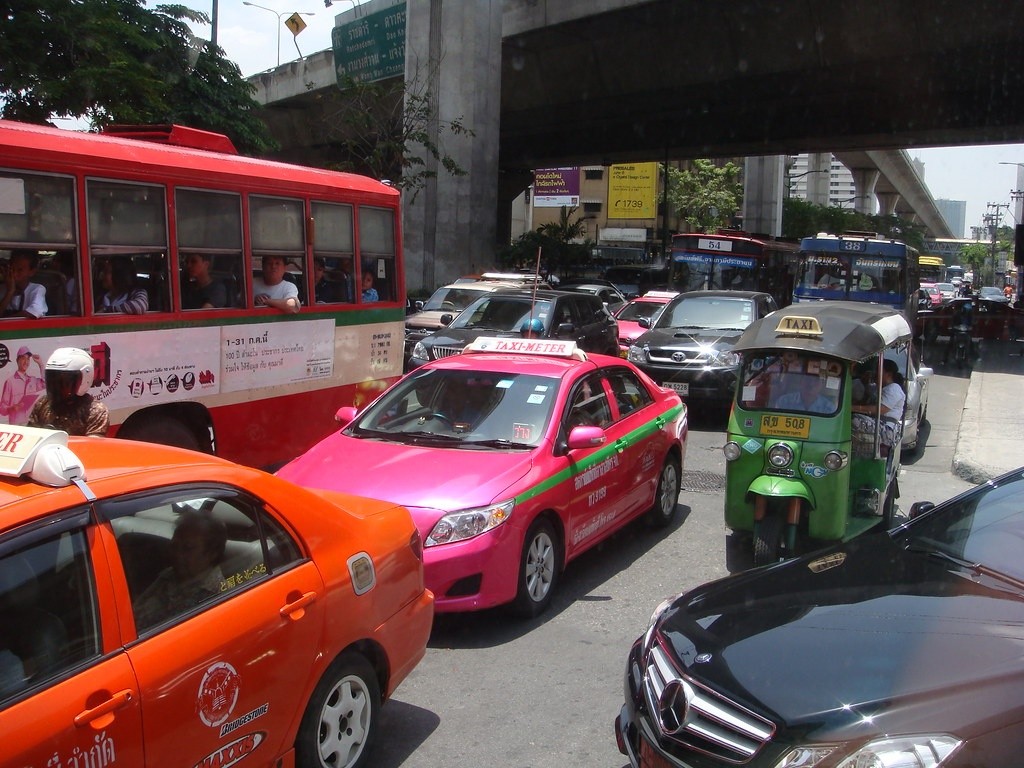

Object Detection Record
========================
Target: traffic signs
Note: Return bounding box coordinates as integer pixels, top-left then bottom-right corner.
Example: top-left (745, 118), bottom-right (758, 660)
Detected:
top-left (331, 2), bottom-right (405, 92)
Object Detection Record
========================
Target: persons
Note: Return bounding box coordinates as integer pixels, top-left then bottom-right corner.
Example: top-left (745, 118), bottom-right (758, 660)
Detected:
top-left (179, 252), bottom-right (220, 308)
top-left (963, 285), bottom-right (973, 298)
top-left (315, 257), bottom-right (335, 303)
top-left (553, 423), bottom-right (568, 454)
top-left (938, 305), bottom-right (981, 366)
top-left (97, 256), bottom-right (148, 315)
top-left (0, 346), bottom-right (46, 428)
top-left (0, 647), bottom-right (37, 705)
top-left (27, 347), bottom-right (109, 438)
top-left (519, 318), bottom-right (544, 339)
top-left (1003, 283), bottom-right (1012, 298)
top-left (440, 384), bottom-right (481, 427)
top-left (0, 244), bottom-right (248, 322)
top-left (255, 255), bottom-right (298, 312)
top-left (850, 359), bottom-right (905, 447)
top-left (773, 370), bottom-right (835, 415)
top-left (362, 268), bottom-right (379, 302)
top-left (0, 250), bottom-right (48, 319)
top-left (739, 352), bottom-right (906, 455)
top-left (131, 511), bottom-right (230, 628)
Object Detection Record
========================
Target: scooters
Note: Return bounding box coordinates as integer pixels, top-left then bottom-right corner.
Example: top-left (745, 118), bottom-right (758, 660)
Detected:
top-left (1003, 292), bottom-right (1012, 302)
top-left (947, 325), bottom-right (973, 370)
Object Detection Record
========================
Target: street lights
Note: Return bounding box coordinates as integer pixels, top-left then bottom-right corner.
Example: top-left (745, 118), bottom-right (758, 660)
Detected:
top-left (243, 2), bottom-right (316, 66)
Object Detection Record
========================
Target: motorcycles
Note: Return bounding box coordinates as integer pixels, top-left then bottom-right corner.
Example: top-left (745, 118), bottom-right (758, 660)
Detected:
top-left (724, 299), bottom-right (913, 567)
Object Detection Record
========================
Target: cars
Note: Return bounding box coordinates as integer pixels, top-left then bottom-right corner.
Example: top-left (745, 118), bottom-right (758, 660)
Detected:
top-left (614, 297), bottom-right (672, 358)
top-left (273, 353), bottom-right (688, 620)
top-left (626, 290), bottom-right (779, 426)
top-left (614, 466), bottom-right (1024, 768)
top-left (403, 280), bottom-right (554, 374)
top-left (454, 275), bottom-right (500, 285)
top-left (0, 435), bottom-right (436, 768)
top-left (558, 284), bottom-right (630, 314)
top-left (407, 289), bottom-right (619, 408)
top-left (918, 277), bottom-right (1024, 342)
top-left (870, 343), bottom-right (934, 457)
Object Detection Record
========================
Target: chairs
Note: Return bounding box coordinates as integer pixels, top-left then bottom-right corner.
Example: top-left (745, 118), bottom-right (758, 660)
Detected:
top-left (34, 266), bottom-right (356, 317)
top-left (608, 374), bottom-right (635, 414)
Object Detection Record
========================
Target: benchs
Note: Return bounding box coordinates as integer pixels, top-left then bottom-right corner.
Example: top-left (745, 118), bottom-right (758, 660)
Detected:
top-left (58, 519), bottom-right (263, 604)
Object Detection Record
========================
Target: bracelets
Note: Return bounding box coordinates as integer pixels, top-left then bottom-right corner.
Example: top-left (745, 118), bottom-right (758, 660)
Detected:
top-left (859, 404), bottom-right (862, 413)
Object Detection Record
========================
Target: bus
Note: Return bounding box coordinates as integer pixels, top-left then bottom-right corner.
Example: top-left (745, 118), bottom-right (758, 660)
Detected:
top-left (919, 255), bottom-right (963, 284)
top-left (791, 231), bottom-right (920, 344)
top-left (0, 121), bottom-right (406, 472)
top-left (667, 230), bottom-right (801, 309)
